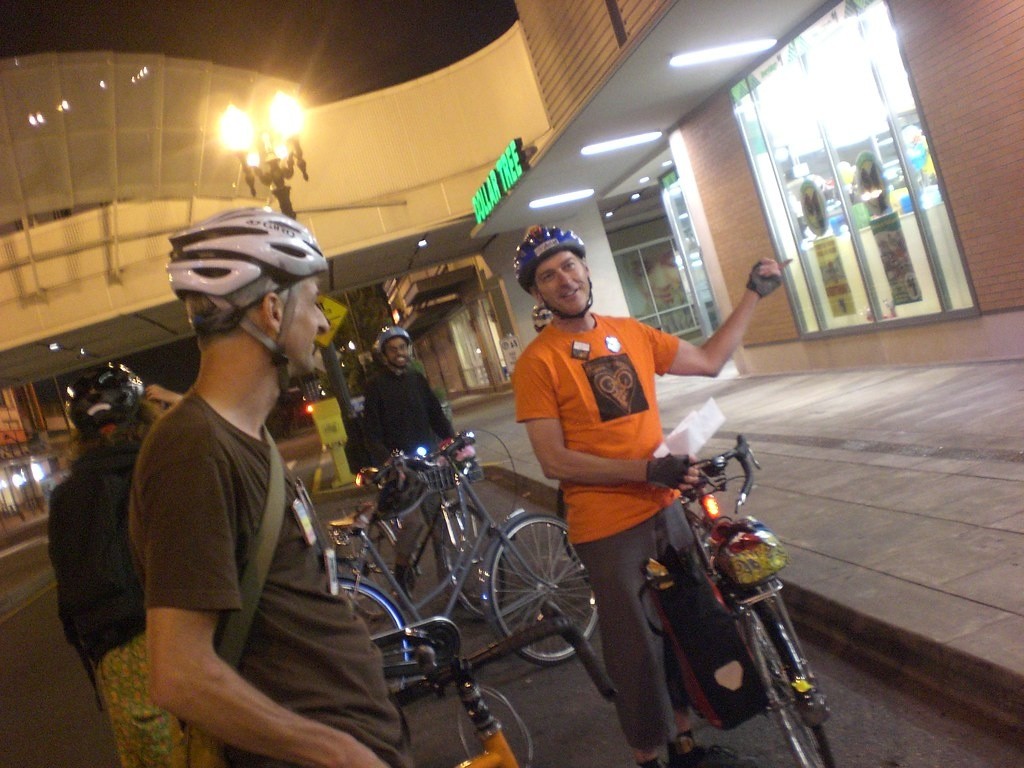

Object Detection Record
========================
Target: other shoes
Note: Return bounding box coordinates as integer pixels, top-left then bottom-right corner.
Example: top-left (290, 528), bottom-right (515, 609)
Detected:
top-left (386, 565), bottom-right (415, 602)
top-left (667, 744), bottom-right (761, 768)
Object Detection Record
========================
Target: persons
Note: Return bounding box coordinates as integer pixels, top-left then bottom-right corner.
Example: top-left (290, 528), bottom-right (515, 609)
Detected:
top-left (363, 327), bottom-right (457, 605)
top-left (513, 224), bottom-right (793, 768)
top-left (131, 209), bottom-right (413, 768)
top-left (50, 364), bottom-right (183, 768)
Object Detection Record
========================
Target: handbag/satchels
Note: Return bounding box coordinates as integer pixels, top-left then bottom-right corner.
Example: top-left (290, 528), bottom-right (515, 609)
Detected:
top-left (80, 594), bottom-right (232, 767)
top-left (639, 544), bottom-right (771, 732)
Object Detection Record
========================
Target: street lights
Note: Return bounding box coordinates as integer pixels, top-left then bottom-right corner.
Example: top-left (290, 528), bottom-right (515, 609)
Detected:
top-left (218, 89), bottom-right (376, 465)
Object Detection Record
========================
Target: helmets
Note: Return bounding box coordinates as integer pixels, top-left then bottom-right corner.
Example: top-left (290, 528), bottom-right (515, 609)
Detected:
top-left (165, 205), bottom-right (330, 315)
top-left (58, 362), bottom-right (146, 434)
top-left (378, 326), bottom-right (411, 349)
top-left (512, 223), bottom-right (587, 288)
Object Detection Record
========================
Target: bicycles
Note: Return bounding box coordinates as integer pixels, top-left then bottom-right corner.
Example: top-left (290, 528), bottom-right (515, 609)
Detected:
top-left (325, 427), bottom-right (839, 768)
